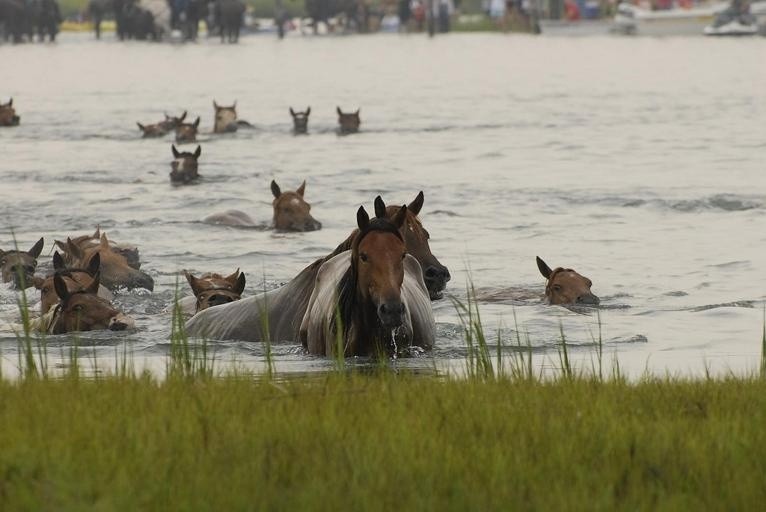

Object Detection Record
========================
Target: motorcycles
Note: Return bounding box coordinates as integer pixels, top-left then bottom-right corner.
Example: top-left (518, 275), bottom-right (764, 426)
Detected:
top-left (697, 10), bottom-right (758, 37)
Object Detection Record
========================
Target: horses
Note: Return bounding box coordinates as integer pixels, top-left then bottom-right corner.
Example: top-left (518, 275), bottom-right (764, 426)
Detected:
top-left (172, 188), bottom-right (451, 345)
top-left (336, 105), bottom-right (361, 136)
top-left (200, 179), bottom-right (323, 233)
top-left (169, 143), bottom-right (202, 188)
top-left (535, 255), bottom-right (601, 307)
top-left (0, 223), bottom-right (248, 336)
top-left (296, 204), bottom-right (437, 357)
top-left (289, 105), bottom-right (311, 137)
top-left (0, 97), bottom-right (21, 127)
top-left (135, 97), bottom-right (251, 146)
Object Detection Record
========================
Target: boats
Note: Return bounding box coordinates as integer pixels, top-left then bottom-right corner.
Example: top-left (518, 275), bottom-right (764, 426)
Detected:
top-left (608, 3), bottom-right (766, 37)
top-left (536, 17), bottom-right (622, 34)
